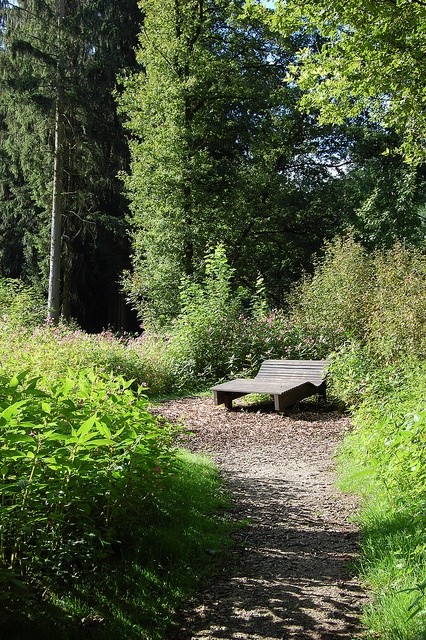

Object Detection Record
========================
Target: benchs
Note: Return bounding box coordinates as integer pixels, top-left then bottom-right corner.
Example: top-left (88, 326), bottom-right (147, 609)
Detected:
top-left (211, 356), bottom-right (334, 413)
